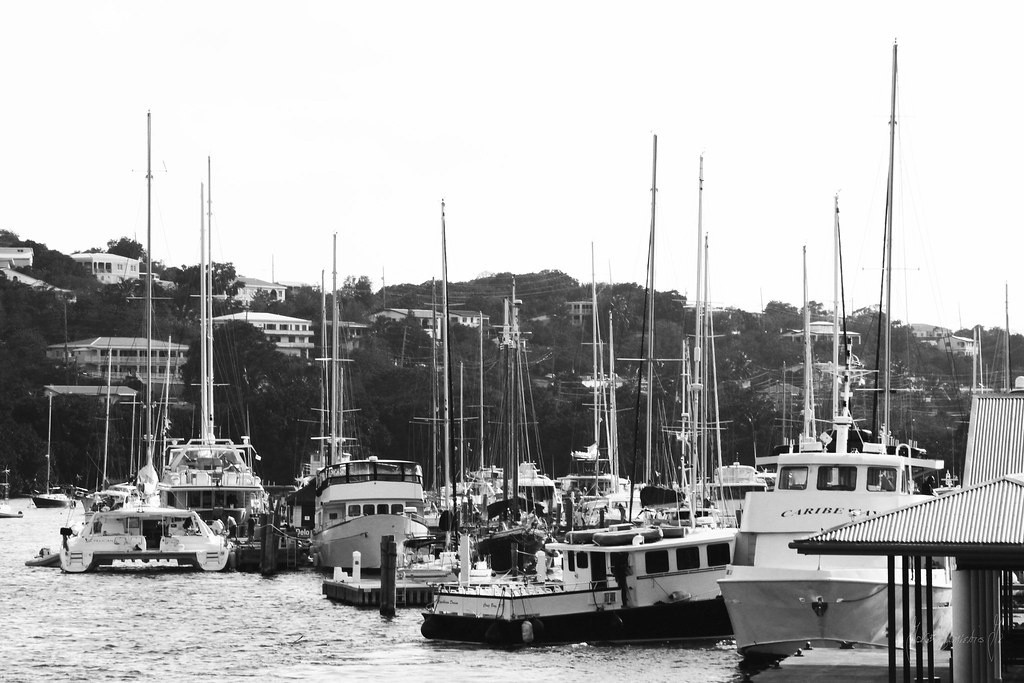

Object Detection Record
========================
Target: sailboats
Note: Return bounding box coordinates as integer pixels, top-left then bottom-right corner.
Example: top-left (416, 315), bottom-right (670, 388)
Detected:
top-left (714, 460), bottom-right (776, 527)
top-left (418, 196), bottom-right (489, 507)
top-left (420, 131), bottom-right (734, 641)
top-left (490, 272), bottom-right (557, 506)
top-left (160, 152), bottom-right (283, 570)
top-left (769, 242), bottom-right (888, 492)
top-left (560, 239), bottom-right (631, 493)
top-left (714, 41), bottom-right (1022, 663)
top-left (670, 337), bottom-right (715, 507)
top-left (285, 234), bottom-right (436, 575)
top-left (60, 110), bottom-right (234, 573)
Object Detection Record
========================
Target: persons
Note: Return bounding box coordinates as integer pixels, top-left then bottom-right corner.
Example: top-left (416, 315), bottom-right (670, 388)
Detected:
top-left (414, 463), bottom-right (423, 486)
top-left (226, 514), bottom-right (238, 542)
top-left (278, 518), bottom-right (288, 532)
top-left (245, 514), bottom-right (256, 544)
top-left (918, 475), bottom-right (935, 496)
